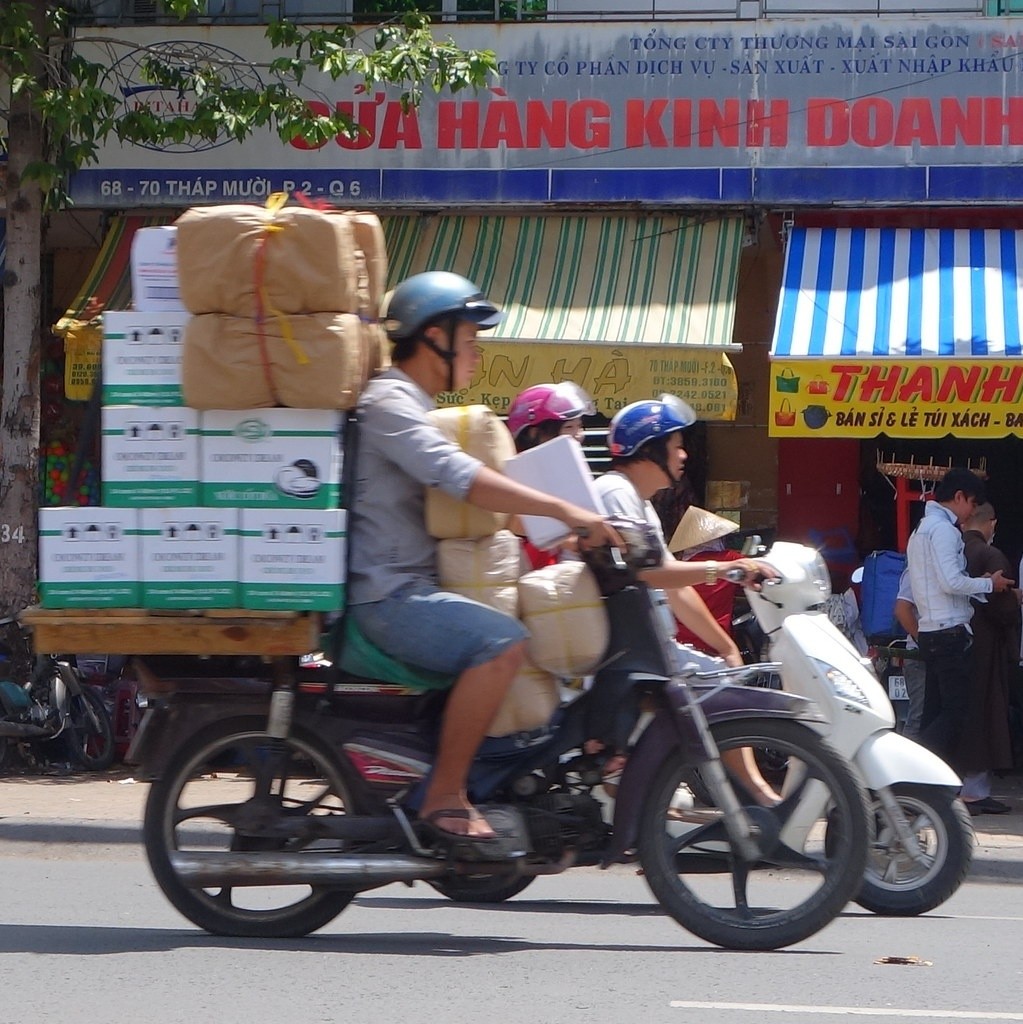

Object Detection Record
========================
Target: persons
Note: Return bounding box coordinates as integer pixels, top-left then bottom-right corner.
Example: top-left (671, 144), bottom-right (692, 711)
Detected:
top-left (894, 469), bottom-right (1023, 817)
top-left (594, 394), bottom-right (779, 805)
top-left (510, 382), bottom-right (597, 571)
top-left (349, 270), bottom-right (623, 844)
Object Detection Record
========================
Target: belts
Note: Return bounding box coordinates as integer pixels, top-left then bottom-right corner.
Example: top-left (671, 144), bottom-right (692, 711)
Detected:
top-left (930, 626), bottom-right (969, 634)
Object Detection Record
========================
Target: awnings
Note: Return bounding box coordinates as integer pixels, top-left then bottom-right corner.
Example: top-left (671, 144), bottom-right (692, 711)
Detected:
top-left (766, 226), bottom-right (1023, 438)
top-left (383, 213), bottom-right (746, 420)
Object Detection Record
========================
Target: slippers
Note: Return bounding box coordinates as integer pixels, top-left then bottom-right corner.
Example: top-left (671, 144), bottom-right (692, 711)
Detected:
top-left (409, 808), bottom-right (499, 845)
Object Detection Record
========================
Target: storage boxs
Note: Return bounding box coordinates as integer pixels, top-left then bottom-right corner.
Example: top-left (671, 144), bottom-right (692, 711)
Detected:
top-left (39, 222), bottom-right (351, 612)
top-left (504, 436), bottom-right (605, 548)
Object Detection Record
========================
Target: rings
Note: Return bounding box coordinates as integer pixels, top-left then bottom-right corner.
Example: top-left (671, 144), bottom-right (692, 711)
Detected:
top-left (750, 564), bottom-right (758, 571)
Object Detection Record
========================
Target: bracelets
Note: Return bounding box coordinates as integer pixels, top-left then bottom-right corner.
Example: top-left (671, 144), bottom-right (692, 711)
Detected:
top-left (706, 561), bottom-right (717, 585)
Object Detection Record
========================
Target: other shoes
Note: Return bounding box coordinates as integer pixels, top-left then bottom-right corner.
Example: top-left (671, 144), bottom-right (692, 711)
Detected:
top-left (964, 797), bottom-right (1012, 816)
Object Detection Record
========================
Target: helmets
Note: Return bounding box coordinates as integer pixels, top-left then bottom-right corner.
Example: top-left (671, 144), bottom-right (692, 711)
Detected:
top-left (606, 393), bottom-right (698, 456)
top-left (506, 381), bottom-right (598, 441)
top-left (385, 269), bottom-right (508, 343)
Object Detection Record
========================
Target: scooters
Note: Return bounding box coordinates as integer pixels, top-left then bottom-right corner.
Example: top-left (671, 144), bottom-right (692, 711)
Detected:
top-left (0, 614), bottom-right (116, 772)
top-left (122, 509), bottom-right (976, 954)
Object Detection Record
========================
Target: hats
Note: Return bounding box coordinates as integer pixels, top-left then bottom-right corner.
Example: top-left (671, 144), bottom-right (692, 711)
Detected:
top-left (666, 504), bottom-right (740, 554)
